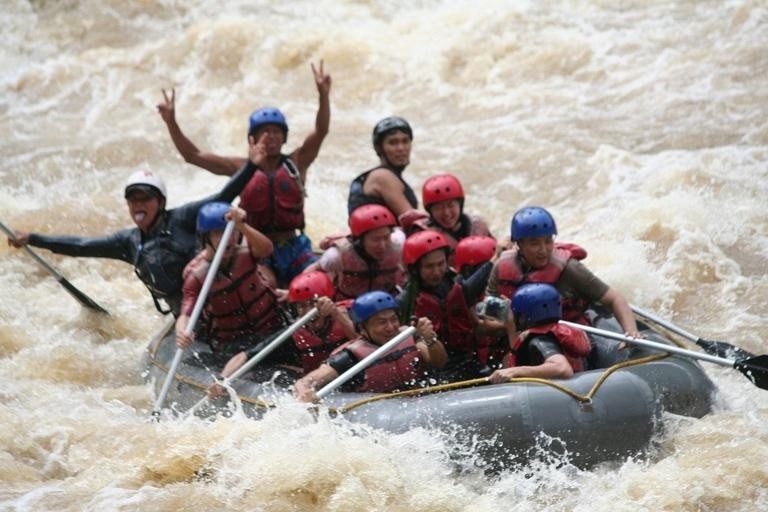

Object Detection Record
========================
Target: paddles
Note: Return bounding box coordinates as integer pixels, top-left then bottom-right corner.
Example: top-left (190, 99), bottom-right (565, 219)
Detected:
top-left (559, 321), bottom-right (766, 389)
top-left (0, 224), bottom-right (108, 311)
top-left (629, 305), bottom-right (756, 360)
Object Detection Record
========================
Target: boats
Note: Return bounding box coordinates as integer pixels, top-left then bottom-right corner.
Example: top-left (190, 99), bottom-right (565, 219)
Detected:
top-left (142, 254), bottom-right (718, 482)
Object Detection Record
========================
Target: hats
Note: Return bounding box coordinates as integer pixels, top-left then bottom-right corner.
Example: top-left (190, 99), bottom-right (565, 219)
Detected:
top-left (125, 185), bottom-right (156, 199)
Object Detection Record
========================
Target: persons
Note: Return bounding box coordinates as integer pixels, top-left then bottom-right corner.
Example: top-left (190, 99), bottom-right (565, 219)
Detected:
top-left (275, 174), bottom-right (498, 404)
top-left (175, 201), bottom-right (280, 362)
top-left (7, 131), bottom-right (271, 320)
top-left (154, 57), bottom-right (334, 255)
top-left (346, 114), bottom-right (420, 222)
top-left (209, 272), bottom-right (360, 400)
top-left (484, 206), bottom-right (645, 383)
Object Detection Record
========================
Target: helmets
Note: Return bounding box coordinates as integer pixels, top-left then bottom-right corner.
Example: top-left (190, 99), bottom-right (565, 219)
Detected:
top-left (288, 273), bottom-right (337, 303)
top-left (248, 106), bottom-right (287, 138)
top-left (196, 203), bottom-right (234, 232)
top-left (510, 284), bottom-right (561, 324)
top-left (373, 116), bottom-right (411, 146)
top-left (511, 207), bottom-right (558, 240)
top-left (402, 176), bottom-right (495, 266)
top-left (124, 171), bottom-right (167, 199)
top-left (350, 291), bottom-right (401, 332)
top-left (349, 204), bottom-right (397, 238)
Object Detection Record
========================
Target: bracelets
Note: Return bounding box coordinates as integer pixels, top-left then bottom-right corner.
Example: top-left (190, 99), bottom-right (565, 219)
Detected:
top-left (218, 377), bottom-right (224, 380)
